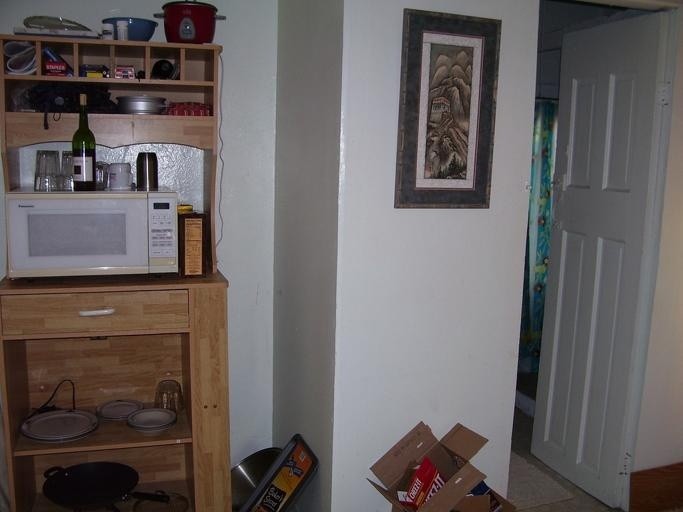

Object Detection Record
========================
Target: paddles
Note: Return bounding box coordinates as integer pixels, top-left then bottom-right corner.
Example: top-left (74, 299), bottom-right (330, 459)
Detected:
top-left (509, 451), bottom-right (576, 511)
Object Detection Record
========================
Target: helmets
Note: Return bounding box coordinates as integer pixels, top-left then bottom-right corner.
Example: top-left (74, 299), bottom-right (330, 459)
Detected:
top-left (19, 399), bottom-right (179, 445)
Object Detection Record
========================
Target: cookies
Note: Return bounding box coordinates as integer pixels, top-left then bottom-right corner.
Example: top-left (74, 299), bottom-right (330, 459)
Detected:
top-left (6, 185), bottom-right (179, 282)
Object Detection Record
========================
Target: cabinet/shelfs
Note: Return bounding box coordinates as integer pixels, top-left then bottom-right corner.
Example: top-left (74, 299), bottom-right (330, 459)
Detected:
top-left (100, 20), bottom-right (129, 40)
top-left (67, 94), bottom-right (100, 193)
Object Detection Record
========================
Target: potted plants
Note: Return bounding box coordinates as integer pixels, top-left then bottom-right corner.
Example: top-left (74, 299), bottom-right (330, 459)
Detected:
top-left (393, 8), bottom-right (501, 209)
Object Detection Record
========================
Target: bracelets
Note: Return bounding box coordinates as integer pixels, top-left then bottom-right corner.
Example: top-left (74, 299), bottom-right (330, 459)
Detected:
top-left (102, 17), bottom-right (159, 41)
top-left (231, 446), bottom-right (285, 509)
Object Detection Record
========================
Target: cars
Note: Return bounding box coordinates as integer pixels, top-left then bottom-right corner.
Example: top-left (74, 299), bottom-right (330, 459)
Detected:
top-left (137, 152), bottom-right (159, 192)
top-left (30, 148), bottom-right (134, 193)
top-left (153, 379), bottom-right (184, 414)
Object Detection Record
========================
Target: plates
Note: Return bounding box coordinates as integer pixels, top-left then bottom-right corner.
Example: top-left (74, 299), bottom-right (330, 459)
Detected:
top-left (0, 32), bottom-right (228, 511)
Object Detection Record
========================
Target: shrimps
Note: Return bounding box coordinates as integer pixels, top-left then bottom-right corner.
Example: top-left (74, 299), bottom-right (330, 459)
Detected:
top-left (153, 0), bottom-right (227, 44)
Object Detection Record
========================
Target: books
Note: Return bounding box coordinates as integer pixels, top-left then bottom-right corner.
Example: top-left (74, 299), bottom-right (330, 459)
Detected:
top-left (365, 419), bottom-right (518, 512)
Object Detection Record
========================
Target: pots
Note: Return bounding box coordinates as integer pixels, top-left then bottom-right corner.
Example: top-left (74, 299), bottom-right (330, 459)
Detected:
top-left (41, 461), bottom-right (173, 510)
